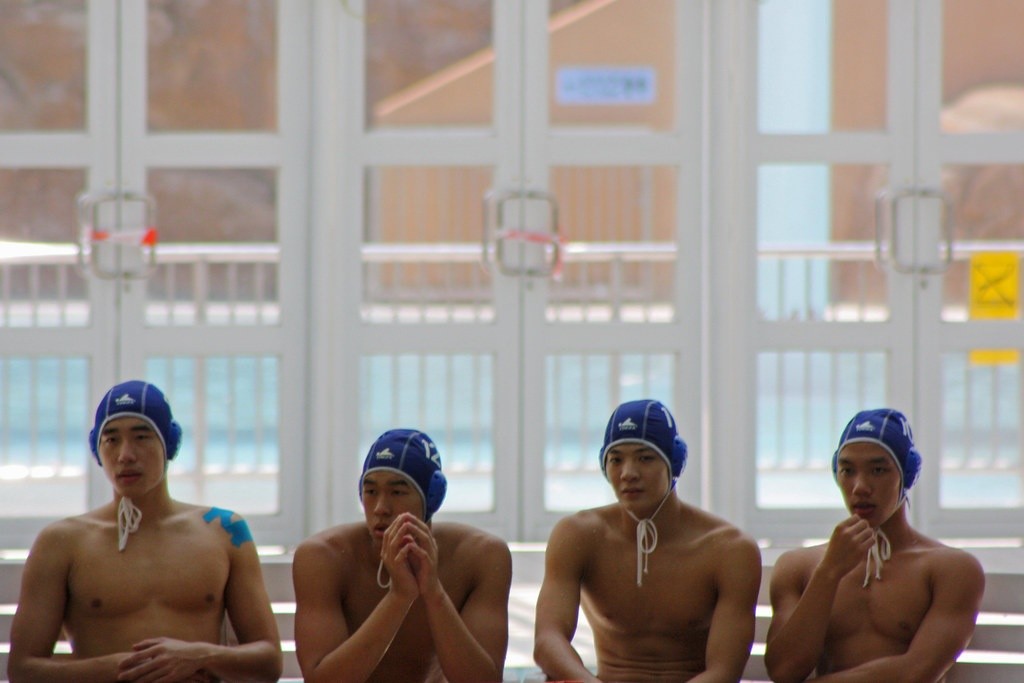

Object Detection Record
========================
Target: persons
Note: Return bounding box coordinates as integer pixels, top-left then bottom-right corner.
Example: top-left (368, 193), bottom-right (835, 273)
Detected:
top-left (765, 408), bottom-right (985, 683)
top-left (533, 399), bottom-right (762, 683)
top-left (6, 380), bottom-right (283, 683)
top-left (292, 429), bottom-right (513, 683)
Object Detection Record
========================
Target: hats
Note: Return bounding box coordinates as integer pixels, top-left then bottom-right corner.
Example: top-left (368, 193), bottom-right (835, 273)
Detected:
top-left (359, 428), bottom-right (448, 524)
top-left (599, 400), bottom-right (688, 493)
top-left (89, 381), bottom-right (183, 466)
top-left (832, 409), bottom-right (921, 509)
